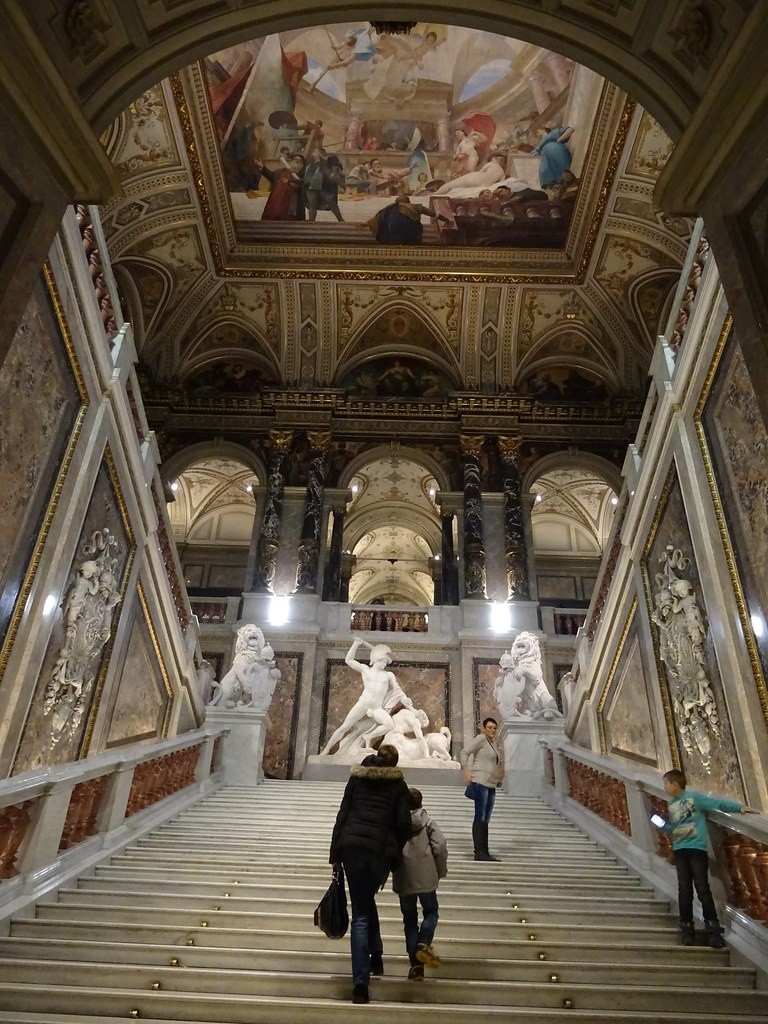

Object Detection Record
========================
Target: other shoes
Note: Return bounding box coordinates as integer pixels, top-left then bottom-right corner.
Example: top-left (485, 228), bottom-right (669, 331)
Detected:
top-left (474, 851), bottom-right (501, 861)
top-left (369, 954), bottom-right (384, 975)
top-left (353, 984), bottom-right (369, 1004)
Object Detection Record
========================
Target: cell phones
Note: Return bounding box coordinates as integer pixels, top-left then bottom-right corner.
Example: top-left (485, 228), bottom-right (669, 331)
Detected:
top-left (651, 814), bottom-right (666, 829)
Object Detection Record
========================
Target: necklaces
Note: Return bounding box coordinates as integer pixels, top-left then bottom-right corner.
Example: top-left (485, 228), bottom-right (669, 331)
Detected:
top-left (486, 736), bottom-right (500, 765)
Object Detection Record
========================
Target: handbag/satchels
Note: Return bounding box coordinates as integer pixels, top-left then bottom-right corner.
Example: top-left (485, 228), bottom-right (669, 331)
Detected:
top-left (314, 866), bottom-right (349, 939)
top-left (464, 784), bottom-right (475, 800)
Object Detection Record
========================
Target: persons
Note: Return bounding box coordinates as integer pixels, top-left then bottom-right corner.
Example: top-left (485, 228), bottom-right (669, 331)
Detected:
top-left (355, 358), bottom-right (440, 397)
top-left (64, 561), bottom-right (101, 627)
top-left (391, 788), bottom-right (448, 983)
top-left (656, 769), bottom-right (759, 948)
top-left (327, 745), bottom-right (411, 1004)
top-left (319, 637), bottom-right (434, 759)
top-left (461, 717), bottom-right (504, 862)
top-left (669, 579), bottom-right (704, 644)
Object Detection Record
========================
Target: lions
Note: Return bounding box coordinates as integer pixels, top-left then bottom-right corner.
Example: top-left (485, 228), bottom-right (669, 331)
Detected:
top-left (208, 624), bottom-right (265, 709)
top-left (510, 630), bottom-right (563, 720)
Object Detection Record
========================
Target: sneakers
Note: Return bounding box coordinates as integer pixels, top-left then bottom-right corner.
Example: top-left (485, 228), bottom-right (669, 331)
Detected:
top-left (679, 921), bottom-right (696, 946)
top-left (415, 943), bottom-right (441, 969)
top-left (705, 919), bottom-right (725, 948)
top-left (408, 965), bottom-right (424, 981)
